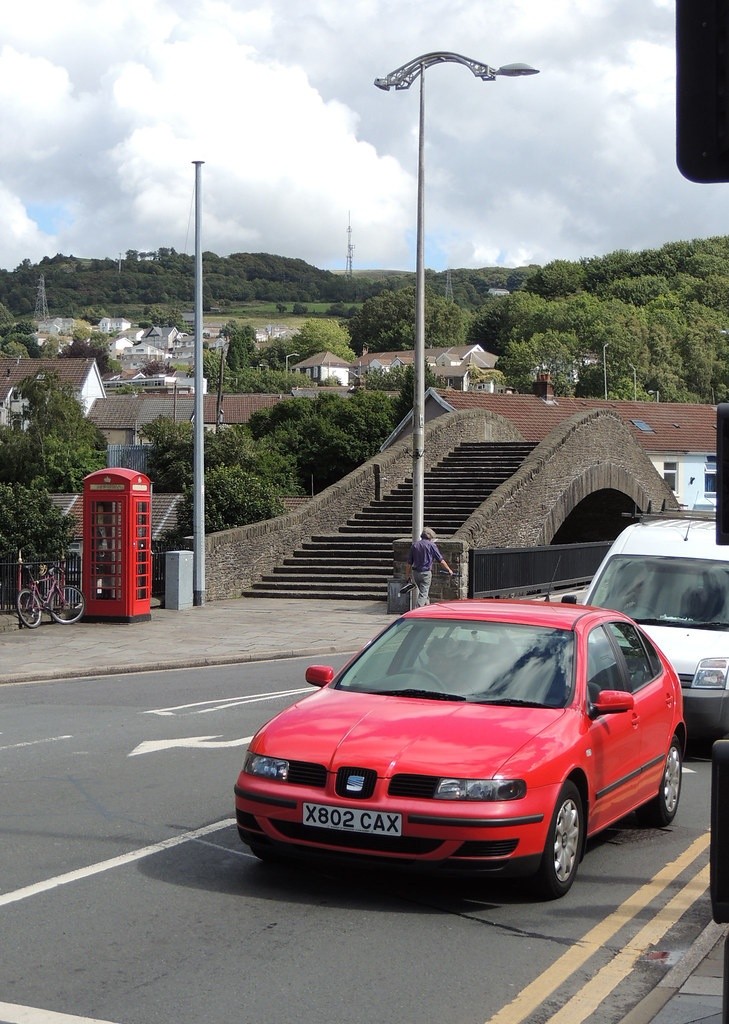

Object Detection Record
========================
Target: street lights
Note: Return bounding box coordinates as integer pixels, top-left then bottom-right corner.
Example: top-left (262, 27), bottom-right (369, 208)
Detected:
top-left (647, 389), bottom-right (660, 403)
top-left (603, 342), bottom-right (611, 401)
top-left (369, 52), bottom-right (542, 542)
top-left (285, 353), bottom-right (300, 380)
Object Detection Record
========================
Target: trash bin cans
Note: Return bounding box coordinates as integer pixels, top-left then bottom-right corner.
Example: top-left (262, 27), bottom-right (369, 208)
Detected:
top-left (385, 577), bottom-right (410, 614)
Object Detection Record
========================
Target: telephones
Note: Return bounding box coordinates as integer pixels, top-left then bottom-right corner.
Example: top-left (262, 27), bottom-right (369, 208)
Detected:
top-left (96, 527), bottom-right (108, 552)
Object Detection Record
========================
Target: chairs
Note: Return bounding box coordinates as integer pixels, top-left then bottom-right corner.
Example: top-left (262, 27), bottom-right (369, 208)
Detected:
top-left (425, 637), bottom-right (470, 678)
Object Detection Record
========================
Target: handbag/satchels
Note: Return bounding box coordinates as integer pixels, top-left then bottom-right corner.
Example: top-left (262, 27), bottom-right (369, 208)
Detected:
top-left (400, 578), bottom-right (415, 595)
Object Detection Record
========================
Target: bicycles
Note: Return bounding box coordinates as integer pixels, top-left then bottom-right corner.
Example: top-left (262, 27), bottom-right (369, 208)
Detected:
top-left (15, 563), bottom-right (87, 628)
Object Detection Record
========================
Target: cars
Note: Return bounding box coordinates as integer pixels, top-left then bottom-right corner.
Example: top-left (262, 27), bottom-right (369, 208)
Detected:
top-left (234, 600), bottom-right (690, 903)
top-left (562, 514), bottom-right (729, 754)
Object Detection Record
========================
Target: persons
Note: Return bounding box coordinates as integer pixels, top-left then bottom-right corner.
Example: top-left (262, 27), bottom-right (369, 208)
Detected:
top-left (405, 526), bottom-right (453, 609)
